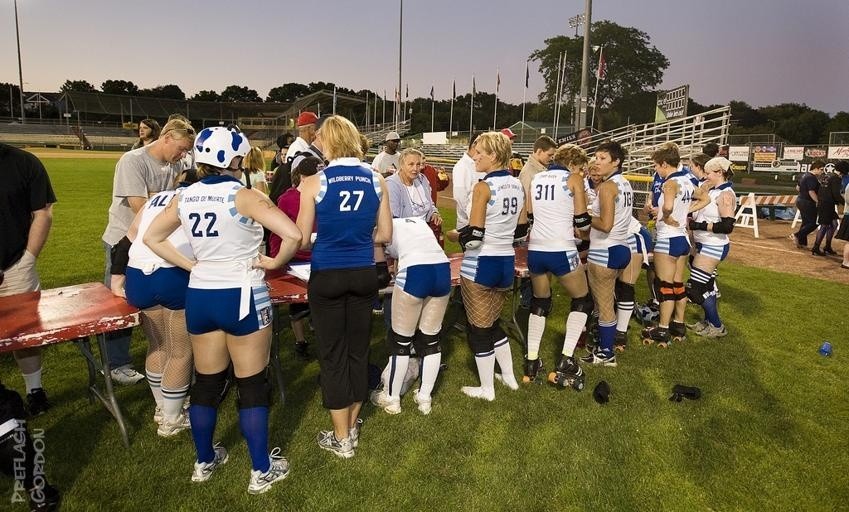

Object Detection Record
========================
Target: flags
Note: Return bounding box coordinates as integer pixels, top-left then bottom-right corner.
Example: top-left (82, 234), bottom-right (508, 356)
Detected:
top-left (473, 77), bottom-right (476, 97)
top-left (526, 67), bottom-right (529, 88)
top-left (453, 80), bottom-right (456, 98)
top-left (497, 73), bottom-right (501, 93)
top-left (430, 85), bottom-right (434, 102)
top-left (597, 53), bottom-right (609, 81)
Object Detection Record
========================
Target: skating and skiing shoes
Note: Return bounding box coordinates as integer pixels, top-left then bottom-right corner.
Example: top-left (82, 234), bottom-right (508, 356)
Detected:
top-left (522, 298), bottom-right (727, 392)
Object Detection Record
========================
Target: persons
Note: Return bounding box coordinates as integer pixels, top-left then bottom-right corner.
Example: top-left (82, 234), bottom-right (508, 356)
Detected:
top-left (811, 160), bottom-right (849, 256)
top-left (833, 182), bottom-right (848, 268)
top-left (787, 160), bottom-right (826, 250)
top-left (1, 112), bottom-right (738, 497)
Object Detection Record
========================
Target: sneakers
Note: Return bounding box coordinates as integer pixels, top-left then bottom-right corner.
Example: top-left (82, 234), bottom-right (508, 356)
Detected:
top-left (370, 390), bottom-right (402, 415)
top-left (24, 388), bottom-right (48, 417)
top-left (247, 447), bottom-right (290, 495)
top-left (372, 298), bottom-right (384, 314)
top-left (192, 442), bottom-right (229, 482)
top-left (413, 389), bottom-right (432, 415)
top-left (154, 395), bottom-right (191, 438)
top-left (295, 337), bottom-right (308, 352)
top-left (101, 364), bottom-right (145, 385)
top-left (317, 418), bottom-right (363, 458)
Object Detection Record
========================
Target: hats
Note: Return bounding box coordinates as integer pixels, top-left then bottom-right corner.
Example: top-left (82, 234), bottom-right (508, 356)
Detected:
top-left (297, 112), bottom-right (318, 126)
top-left (500, 128), bottom-right (518, 138)
top-left (386, 132), bottom-right (402, 142)
top-left (298, 156), bottom-right (325, 175)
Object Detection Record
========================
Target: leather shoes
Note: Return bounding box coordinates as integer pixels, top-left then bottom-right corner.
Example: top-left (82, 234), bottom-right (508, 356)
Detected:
top-left (794, 232), bottom-right (807, 245)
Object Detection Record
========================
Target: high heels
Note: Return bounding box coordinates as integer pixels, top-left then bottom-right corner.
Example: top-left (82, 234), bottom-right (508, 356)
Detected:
top-left (812, 247), bottom-right (825, 256)
top-left (823, 246), bottom-right (836, 254)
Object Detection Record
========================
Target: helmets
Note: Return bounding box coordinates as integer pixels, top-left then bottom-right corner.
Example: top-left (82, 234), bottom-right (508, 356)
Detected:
top-left (193, 125), bottom-right (252, 169)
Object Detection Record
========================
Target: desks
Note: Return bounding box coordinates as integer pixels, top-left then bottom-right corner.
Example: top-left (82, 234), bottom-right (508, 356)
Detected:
top-left (264, 242), bottom-right (656, 407)
top-left (0, 280), bottom-right (142, 447)
